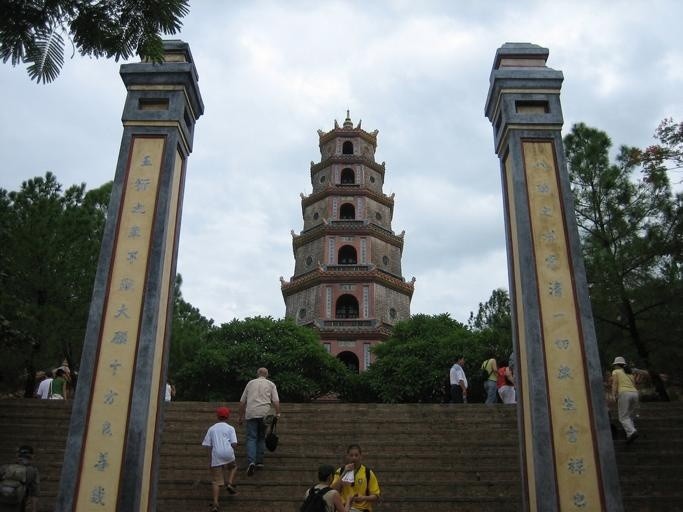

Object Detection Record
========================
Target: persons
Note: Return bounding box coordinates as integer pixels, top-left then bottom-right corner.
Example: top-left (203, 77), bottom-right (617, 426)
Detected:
top-left (448, 348), bottom-right (516, 405)
top-left (330, 443), bottom-right (381, 512)
top-left (201, 406), bottom-right (239, 512)
top-left (163, 379), bottom-right (177, 402)
top-left (303, 464), bottom-right (355, 512)
top-left (46, 369), bottom-right (68, 399)
top-left (36, 370), bottom-right (54, 400)
top-left (611, 355), bottom-right (641, 440)
top-left (0, 444), bottom-right (42, 510)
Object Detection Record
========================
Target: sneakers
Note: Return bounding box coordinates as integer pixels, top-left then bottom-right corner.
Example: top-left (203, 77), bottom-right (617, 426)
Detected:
top-left (211, 504), bottom-right (220, 512)
top-left (247, 463), bottom-right (265, 477)
top-left (227, 483), bottom-right (237, 494)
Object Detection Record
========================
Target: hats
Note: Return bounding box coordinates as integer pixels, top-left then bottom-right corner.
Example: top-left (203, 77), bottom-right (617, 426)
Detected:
top-left (18, 446), bottom-right (34, 454)
top-left (216, 407), bottom-right (229, 418)
top-left (613, 357), bottom-right (627, 365)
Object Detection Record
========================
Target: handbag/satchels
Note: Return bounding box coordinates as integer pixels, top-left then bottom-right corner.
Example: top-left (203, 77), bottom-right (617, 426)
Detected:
top-left (50, 393), bottom-right (64, 399)
top-left (265, 431), bottom-right (279, 453)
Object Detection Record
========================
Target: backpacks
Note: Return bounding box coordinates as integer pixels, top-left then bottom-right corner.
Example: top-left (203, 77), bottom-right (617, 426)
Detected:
top-left (479, 359), bottom-right (493, 383)
top-left (0, 461), bottom-right (31, 506)
top-left (300, 483), bottom-right (332, 512)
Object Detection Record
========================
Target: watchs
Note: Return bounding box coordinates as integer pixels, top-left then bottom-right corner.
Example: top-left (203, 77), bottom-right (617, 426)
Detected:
top-left (235, 367), bottom-right (281, 475)
